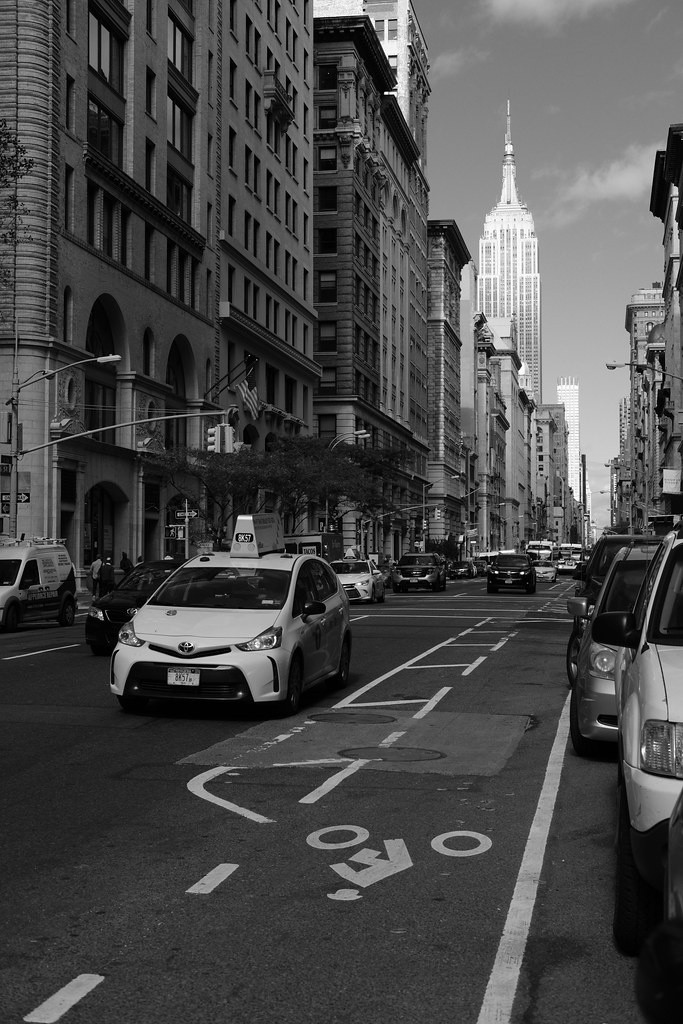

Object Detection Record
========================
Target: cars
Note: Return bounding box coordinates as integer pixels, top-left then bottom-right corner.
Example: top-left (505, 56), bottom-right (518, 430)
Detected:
top-left (85, 559), bottom-right (198, 655)
top-left (565, 533), bottom-right (665, 688)
top-left (447, 561), bottom-right (477, 579)
top-left (109, 552), bottom-right (353, 717)
top-left (331, 559), bottom-right (384, 603)
top-left (533, 560), bottom-right (556, 583)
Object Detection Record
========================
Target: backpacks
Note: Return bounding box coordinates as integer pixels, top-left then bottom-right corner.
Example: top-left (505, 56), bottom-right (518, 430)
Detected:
top-left (100, 565), bottom-right (111, 584)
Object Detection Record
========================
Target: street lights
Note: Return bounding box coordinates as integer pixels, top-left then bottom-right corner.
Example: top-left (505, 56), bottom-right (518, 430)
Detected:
top-left (463, 502), bottom-right (506, 559)
top-left (605, 464), bottom-right (649, 535)
top-left (324, 430), bottom-right (371, 532)
top-left (5, 354), bottom-right (123, 538)
top-left (601, 491), bottom-right (634, 535)
top-left (422, 475), bottom-right (459, 552)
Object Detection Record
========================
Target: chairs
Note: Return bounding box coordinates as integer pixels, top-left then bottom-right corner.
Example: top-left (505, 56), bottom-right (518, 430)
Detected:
top-left (205, 582), bottom-right (244, 607)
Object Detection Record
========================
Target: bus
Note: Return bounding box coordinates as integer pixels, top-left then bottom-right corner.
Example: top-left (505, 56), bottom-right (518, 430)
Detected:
top-left (526, 540), bottom-right (560, 561)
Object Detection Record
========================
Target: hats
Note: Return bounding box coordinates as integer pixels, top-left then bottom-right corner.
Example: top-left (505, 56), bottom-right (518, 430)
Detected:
top-left (96, 554), bottom-right (100, 557)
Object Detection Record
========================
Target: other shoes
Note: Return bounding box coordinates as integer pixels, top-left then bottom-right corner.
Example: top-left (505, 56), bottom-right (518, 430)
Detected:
top-left (92, 595), bottom-right (96, 601)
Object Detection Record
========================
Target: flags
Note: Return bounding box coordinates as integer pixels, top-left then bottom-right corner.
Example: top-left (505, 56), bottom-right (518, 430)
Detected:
top-left (235, 367), bottom-right (259, 421)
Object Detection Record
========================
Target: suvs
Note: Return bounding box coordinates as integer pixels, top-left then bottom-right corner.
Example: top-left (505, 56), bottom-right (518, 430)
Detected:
top-left (487, 552), bottom-right (536, 594)
top-left (0, 533), bottom-right (78, 630)
top-left (392, 553), bottom-right (448, 592)
top-left (566, 540), bottom-right (647, 758)
top-left (593, 510), bottom-right (683, 957)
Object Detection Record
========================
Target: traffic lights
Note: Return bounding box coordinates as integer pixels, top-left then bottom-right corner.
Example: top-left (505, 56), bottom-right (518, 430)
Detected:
top-left (423, 519), bottom-right (427, 531)
top-left (165, 526), bottom-right (176, 539)
top-left (207, 426), bottom-right (220, 453)
top-left (224, 427), bottom-right (236, 454)
top-left (435, 508), bottom-right (441, 520)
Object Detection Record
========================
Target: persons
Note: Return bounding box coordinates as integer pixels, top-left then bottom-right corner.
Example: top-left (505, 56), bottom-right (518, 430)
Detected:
top-left (89, 554), bottom-right (105, 602)
top-left (120, 551), bottom-right (131, 574)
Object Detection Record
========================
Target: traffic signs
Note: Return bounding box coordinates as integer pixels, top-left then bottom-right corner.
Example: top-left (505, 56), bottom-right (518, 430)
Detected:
top-left (1, 503), bottom-right (18, 515)
top-left (1, 492), bottom-right (30, 504)
top-left (175, 509), bottom-right (199, 519)
